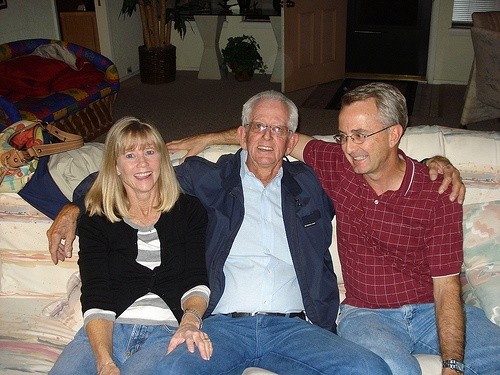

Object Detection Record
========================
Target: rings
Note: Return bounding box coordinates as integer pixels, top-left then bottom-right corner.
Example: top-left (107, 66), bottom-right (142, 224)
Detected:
top-left (203, 337), bottom-right (208, 342)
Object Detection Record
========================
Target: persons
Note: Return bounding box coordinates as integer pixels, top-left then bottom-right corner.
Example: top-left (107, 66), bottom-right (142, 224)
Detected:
top-left (166, 84), bottom-right (500, 375)
top-left (48, 117), bottom-right (213, 374)
top-left (47, 91), bottom-right (394, 375)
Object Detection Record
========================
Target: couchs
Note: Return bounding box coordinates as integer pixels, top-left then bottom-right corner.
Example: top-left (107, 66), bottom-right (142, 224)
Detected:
top-left (0, 37), bottom-right (119, 145)
top-left (1, 124), bottom-right (500, 375)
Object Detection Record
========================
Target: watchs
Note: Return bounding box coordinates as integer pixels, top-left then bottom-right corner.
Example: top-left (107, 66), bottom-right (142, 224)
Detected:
top-left (442, 359), bottom-right (466, 373)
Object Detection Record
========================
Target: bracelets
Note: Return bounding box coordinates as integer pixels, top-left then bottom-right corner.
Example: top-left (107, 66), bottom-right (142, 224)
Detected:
top-left (182, 308), bottom-right (203, 331)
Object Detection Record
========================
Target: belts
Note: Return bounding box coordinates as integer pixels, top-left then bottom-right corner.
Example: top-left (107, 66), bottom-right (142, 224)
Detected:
top-left (227, 312), bottom-right (303, 318)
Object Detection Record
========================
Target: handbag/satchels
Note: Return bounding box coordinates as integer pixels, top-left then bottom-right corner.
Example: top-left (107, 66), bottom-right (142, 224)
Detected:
top-left (0, 118), bottom-right (83, 174)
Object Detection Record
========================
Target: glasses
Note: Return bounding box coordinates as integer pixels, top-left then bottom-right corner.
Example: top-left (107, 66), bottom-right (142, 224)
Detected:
top-left (333, 123), bottom-right (399, 145)
top-left (242, 122), bottom-right (293, 136)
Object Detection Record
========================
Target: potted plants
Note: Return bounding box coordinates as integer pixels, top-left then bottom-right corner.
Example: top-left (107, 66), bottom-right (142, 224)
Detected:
top-left (219, 34), bottom-right (269, 83)
top-left (187, 0), bottom-right (236, 80)
top-left (268, 1), bottom-right (283, 83)
top-left (118, 0), bottom-right (193, 84)
